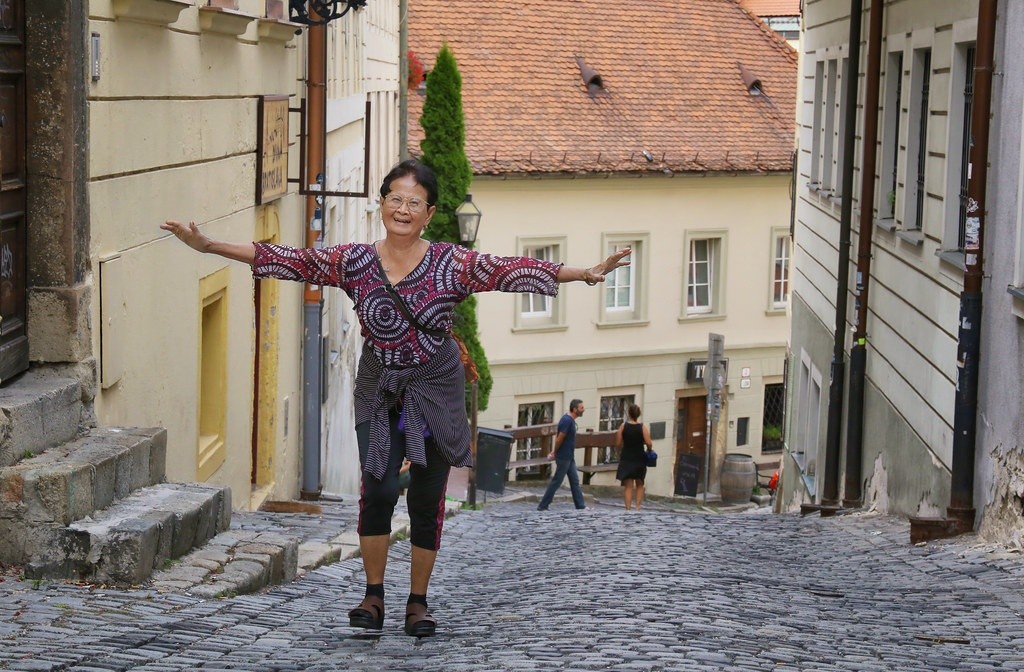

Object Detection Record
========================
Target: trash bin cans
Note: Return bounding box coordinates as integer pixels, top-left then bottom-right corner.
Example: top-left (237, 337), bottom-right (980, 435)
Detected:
top-left (475, 427), bottom-right (514, 493)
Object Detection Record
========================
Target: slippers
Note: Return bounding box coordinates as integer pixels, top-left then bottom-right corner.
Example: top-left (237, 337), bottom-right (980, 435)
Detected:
top-left (405, 602), bottom-right (439, 637)
top-left (348, 597), bottom-right (380, 629)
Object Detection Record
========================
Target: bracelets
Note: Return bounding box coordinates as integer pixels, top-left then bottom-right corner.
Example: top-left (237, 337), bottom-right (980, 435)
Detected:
top-left (583, 267), bottom-right (598, 286)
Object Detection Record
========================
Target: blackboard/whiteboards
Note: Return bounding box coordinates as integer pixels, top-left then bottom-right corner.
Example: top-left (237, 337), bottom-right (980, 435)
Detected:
top-left (674, 453), bottom-right (702, 498)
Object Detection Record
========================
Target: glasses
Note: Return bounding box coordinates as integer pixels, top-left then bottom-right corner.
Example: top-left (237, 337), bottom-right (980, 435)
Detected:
top-left (383, 195), bottom-right (429, 213)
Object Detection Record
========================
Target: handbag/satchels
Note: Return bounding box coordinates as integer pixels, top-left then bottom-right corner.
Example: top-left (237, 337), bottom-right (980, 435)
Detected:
top-left (451, 330), bottom-right (480, 383)
top-left (644, 450), bottom-right (657, 467)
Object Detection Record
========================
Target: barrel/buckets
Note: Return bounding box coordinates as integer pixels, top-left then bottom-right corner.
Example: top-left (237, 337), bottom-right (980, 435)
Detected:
top-left (721, 453), bottom-right (756, 504)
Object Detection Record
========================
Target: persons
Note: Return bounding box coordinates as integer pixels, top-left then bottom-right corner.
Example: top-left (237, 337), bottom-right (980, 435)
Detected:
top-left (398, 457), bottom-right (411, 488)
top-left (616, 404), bottom-right (652, 511)
top-left (159, 160), bottom-right (632, 636)
top-left (537, 399), bottom-right (590, 510)
